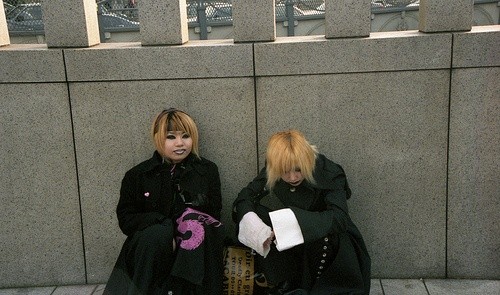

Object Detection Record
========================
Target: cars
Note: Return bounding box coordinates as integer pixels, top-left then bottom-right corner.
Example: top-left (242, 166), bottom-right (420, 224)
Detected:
top-left (0, 0), bottom-right (422, 34)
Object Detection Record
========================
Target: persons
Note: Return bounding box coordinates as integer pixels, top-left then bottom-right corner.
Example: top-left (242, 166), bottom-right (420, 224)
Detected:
top-left (231, 129), bottom-right (371, 295)
top-left (103, 108), bottom-right (224, 295)
